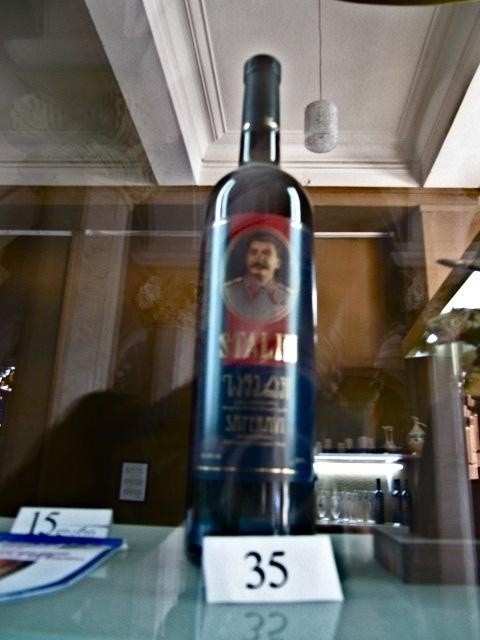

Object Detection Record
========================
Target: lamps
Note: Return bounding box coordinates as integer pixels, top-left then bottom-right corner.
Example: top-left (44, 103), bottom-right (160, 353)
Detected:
top-left (301, 0), bottom-right (342, 153)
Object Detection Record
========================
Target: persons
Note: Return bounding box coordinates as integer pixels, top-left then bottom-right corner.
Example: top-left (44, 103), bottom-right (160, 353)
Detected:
top-left (225, 235), bottom-right (285, 318)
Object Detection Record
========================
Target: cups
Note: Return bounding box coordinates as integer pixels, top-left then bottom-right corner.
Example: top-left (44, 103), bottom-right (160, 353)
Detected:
top-left (316, 489), bottom-right (374, 524)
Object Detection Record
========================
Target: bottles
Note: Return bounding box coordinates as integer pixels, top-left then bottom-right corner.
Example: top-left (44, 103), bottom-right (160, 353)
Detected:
top-left (185, 52), bottom-right (316, 568)
top-left (373, 478), bottom-right (385, 525)
top-left (391, 478), bottom-right (413, 527)
